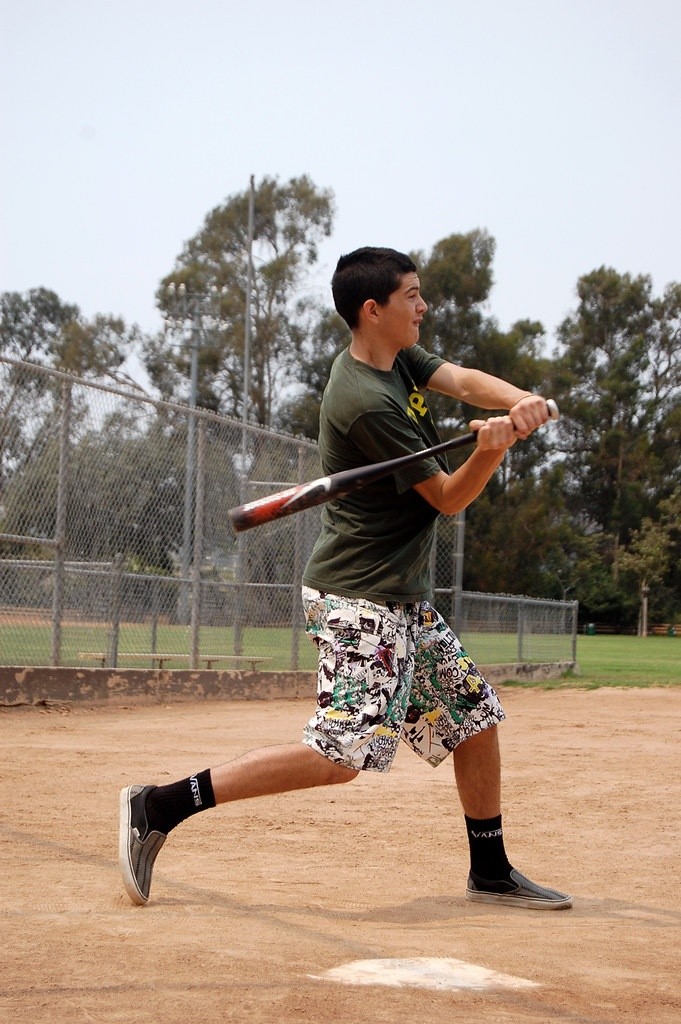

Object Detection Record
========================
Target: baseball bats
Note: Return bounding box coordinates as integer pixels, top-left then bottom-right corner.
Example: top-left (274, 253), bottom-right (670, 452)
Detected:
top-left (227, 397), bottom-right (562, 535)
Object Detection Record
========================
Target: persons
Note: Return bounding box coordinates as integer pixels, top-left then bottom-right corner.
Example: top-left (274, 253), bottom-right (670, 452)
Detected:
top-left (118, 247), bottom-right (574, 909)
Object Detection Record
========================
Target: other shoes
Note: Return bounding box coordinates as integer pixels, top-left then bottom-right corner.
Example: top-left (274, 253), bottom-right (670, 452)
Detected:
top-left (466, 867), bottom-right (573, 910)
top-left (119, 783), bottom-right (168, 906)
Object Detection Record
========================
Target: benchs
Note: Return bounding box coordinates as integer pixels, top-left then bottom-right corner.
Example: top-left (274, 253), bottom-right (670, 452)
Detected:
top-left (80, 652), bottom-right (274, 670)
